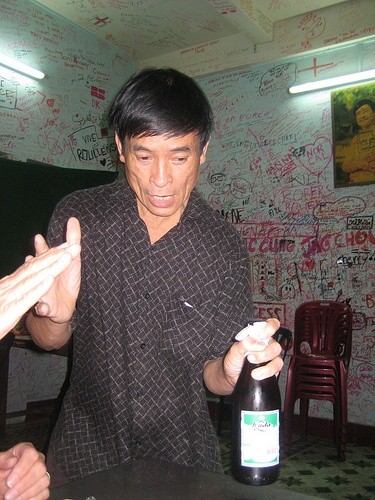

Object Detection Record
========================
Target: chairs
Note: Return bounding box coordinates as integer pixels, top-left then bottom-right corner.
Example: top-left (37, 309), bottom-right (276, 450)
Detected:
top-left (284, 300), bottom-right (354, 461)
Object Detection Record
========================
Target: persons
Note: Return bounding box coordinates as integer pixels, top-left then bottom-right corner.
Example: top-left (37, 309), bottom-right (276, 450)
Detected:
top-left (0, 242), bottom-right (81, 500)
top-left (25, 67), bottom-right (284, 488)
top-left (341, 99), bottom-right (375, 183)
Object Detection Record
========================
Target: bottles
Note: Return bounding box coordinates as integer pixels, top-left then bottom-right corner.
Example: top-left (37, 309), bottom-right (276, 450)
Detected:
top-left (232, 319), bottom-right (281, 486)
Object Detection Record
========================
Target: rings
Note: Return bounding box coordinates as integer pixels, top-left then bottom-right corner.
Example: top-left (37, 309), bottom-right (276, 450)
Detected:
top-left (46, 472), bottom-right (51, 479)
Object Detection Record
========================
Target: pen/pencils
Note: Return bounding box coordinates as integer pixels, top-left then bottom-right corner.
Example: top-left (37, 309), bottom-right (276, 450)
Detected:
top-left (178, 293), bottom-right (193, 308)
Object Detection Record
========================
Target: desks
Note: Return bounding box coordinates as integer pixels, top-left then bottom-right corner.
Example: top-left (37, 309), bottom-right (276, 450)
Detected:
top-left (45, 456), bottom-right (324, 500)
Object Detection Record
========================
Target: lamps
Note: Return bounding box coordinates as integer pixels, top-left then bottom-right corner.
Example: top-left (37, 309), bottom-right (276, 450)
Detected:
top-left (1, 53), bottom-right (46, 83)
top-left (288, 69), bottom-right (375, 95)
